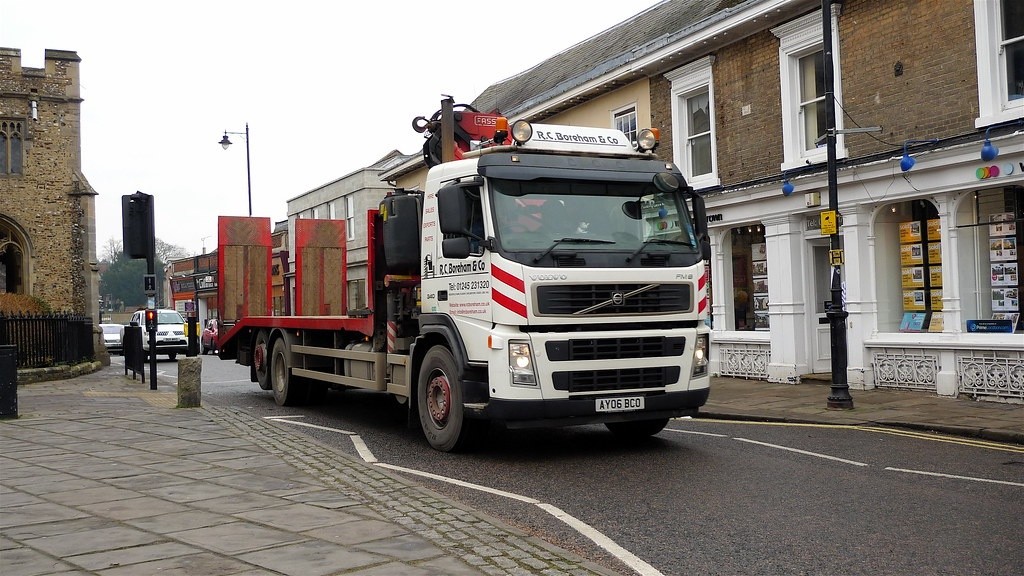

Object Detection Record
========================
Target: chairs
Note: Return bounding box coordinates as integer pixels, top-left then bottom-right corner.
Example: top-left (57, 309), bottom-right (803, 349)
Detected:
top-left (468, 200), bottom-right (505, 241)
top-left (534, 200), bottom-right (576, 235)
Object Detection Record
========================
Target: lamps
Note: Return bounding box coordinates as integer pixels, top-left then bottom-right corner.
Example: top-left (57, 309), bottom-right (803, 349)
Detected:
top-left (900, 138), bottom-right (937, 171)
top-left (782, 166), bottom-right (815, 197)
top-left (981, 121), bottom-right (1024, 161)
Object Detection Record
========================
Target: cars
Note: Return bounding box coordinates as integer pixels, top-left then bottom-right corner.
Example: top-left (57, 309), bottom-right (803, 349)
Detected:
top-left (200, 318), bottom-right (219, 353)
top-left (98, 323), bottom-right (124, 349)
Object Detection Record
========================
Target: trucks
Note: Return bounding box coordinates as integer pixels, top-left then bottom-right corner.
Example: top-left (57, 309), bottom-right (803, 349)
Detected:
top-left (217, 93), bottom-right (711, 453)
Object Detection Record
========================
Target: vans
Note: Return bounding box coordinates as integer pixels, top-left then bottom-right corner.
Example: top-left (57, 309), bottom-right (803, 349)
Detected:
top-left (130, 308), bottom-right (188, 360)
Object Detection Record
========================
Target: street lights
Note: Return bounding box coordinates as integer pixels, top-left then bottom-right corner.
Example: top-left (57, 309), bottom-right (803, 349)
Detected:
top-left (217, 122), bottom-right (252, 216)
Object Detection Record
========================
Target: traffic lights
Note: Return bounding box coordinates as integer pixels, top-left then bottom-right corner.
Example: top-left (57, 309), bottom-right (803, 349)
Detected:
top-left (145, 310), bottom-right (158, 331)
